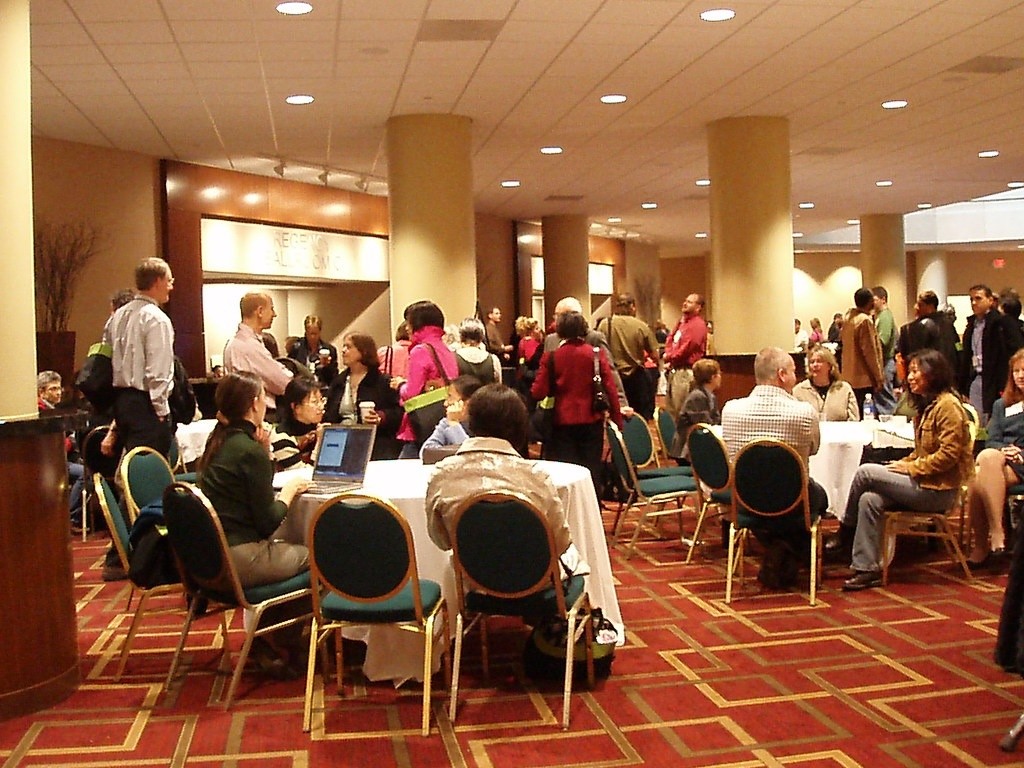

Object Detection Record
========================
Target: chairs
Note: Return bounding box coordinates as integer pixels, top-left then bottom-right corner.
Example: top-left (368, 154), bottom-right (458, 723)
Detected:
top-left (447, 489), bottom-right (595, 732)
top-left (162, 481), bottom-right (330, 714)
top-left (304, 497), bottom-right (453, 738)
top-left (82, 423), bottom-right (238, 681)
top-left (606, 403), bottom-right (1024, 609)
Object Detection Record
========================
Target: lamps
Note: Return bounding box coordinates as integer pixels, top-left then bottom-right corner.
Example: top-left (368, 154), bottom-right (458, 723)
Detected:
top-left (621, 229), bottom-right (628, 239)
top-left (318, 166), bottom-right (331, 184)
top-left (273, 160), bottom-right (288, 178)
top-left (605, 226), bottom-right (613, 235)
top-left (354, 173), bottom-right (368, 189)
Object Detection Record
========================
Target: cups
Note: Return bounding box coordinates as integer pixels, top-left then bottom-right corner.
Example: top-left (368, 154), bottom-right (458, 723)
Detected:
top-left (319, 349), bottom-right (330, 365)
top-left (360, 402), bottom-right (376, 422)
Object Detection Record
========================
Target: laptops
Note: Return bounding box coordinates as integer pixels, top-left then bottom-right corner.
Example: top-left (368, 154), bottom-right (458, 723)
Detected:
top-left (274, 425), bottom-right (377, 494)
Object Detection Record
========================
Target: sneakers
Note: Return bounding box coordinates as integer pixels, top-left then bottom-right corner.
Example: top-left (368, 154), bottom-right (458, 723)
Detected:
top-left (826, 521), bottom-right (856, 552)
top-left (842, 570), bottom-right (881, 589)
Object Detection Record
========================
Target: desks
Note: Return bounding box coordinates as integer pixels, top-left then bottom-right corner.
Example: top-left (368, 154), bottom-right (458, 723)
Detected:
top-left (699, 420), bottom-right (975, 556)
top-left (175, 418), bottom-right (220, 467)
top-left (272, 458), bottom-right (625, 690)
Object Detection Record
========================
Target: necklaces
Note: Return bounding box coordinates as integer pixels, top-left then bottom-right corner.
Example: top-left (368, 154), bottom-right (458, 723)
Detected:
top-left (821, 393), bottom-right (826, 399)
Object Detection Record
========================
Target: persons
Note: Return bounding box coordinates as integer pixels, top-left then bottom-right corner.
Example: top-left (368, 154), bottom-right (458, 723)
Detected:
top-left (721, 346), bottom-right (821, 593)
top-left (37, 371), bottom-right (89, 532)
top-left (102, 256), bottom-right (174, 580)
top-left (822, 348), bottom-right (971, 588)
top-left (967, 349), bottom-right (1024, 571)
top-left (195, 370), bottom-right (320, 683)
top-left (211, 293), bottom-right (721, 517)
top-left (424, 385), bottom-right (573, 591)
top-left (792, 285), bottom-right (1024, 428)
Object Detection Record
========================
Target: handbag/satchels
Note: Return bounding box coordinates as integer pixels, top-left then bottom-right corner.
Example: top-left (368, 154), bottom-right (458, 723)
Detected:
top-left (593, 347), bottom-right (610, 411)
top-left (525, 608), bottom-right (618, 682)
top-left (79, 353), bottom-right (116, 414)
top-left (400, 344), bottom-right (451, 450)
top-left (534, 401), bottom-right (555, 441)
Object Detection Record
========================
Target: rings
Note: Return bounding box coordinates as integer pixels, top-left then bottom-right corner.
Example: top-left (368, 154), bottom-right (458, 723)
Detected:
top-left (378, 417), bottom-right (381, 422)
top-left (1017, 455), bottom-right (1019, 460)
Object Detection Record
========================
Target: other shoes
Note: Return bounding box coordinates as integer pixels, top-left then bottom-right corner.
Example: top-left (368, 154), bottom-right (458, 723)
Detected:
top-left (966, 552), bottom-right (991, 570)
top-left (757, 549), bottom-right (799, 588)
top-left (70, 526), bottom-right (90, 532)
top-left (103, 566), bottom-right (127, 581)
top-left (256, 654), bottom-right (298, 681)
top-left (990, 532), bottom-right (1007, 557)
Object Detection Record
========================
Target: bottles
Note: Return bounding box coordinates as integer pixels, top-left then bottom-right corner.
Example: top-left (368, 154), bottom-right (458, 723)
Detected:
top-left (863, 393), bottom-right (874, 422)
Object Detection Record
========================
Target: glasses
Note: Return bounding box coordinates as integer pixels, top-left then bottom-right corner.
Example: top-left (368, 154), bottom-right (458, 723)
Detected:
top-left (301, 397), bottom-right (327, 408)
top-left (167, 278), bottom-right (174, 283)
top-left (49, 387), bottom-right (65, 393)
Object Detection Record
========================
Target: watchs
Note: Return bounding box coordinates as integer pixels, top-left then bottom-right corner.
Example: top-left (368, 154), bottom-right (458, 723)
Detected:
top-left (110, 423), bottom-right (119, 432)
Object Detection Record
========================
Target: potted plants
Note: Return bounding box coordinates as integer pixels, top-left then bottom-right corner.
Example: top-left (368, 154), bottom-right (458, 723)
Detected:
top-left (34, 219), bottom-right (100, 388)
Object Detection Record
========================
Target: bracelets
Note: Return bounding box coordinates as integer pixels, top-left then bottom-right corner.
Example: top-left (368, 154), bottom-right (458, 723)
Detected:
top-left (396, 381), bottom-right (407, 396)
